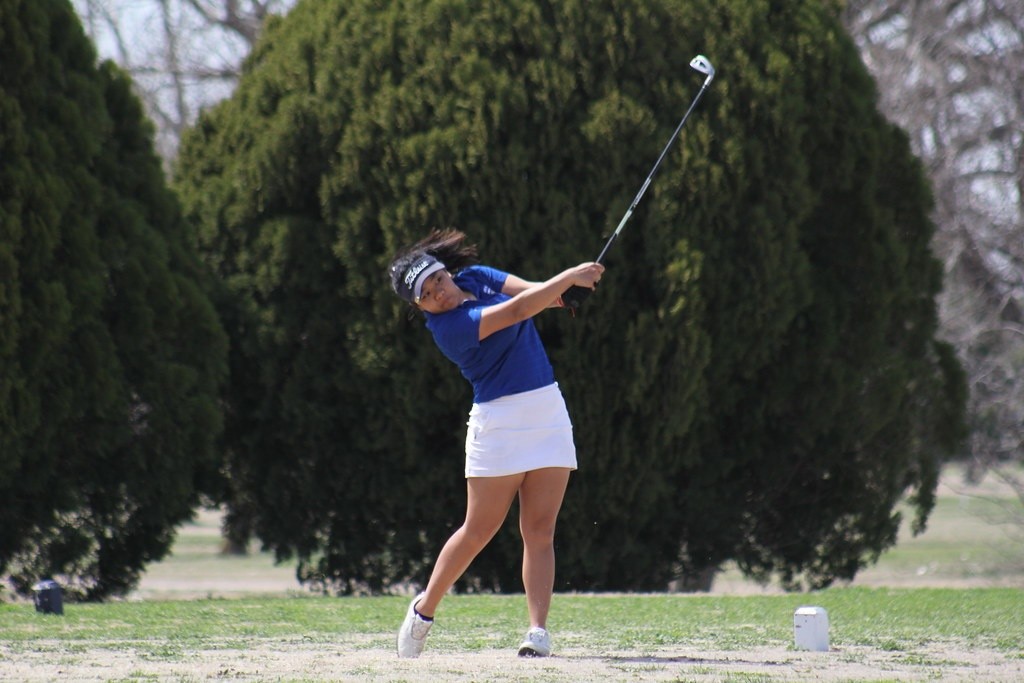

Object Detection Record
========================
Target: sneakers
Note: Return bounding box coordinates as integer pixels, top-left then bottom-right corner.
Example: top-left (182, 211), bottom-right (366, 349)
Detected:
top-left (518, 626), bottom-right (552, 657)
top-left (397, 591), bottom-right (435, 658)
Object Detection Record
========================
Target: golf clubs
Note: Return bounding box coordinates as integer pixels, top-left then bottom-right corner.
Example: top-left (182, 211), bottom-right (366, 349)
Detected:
top-left (594, 53), bottom-right (716, 268)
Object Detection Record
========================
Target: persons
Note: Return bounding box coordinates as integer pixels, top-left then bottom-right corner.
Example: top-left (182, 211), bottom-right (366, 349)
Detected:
top-left (389, 229), bottom-right (605, 660)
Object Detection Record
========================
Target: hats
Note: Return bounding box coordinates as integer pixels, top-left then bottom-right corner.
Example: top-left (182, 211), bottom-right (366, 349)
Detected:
top-left (398, 255), bottom-right (446, 304)
